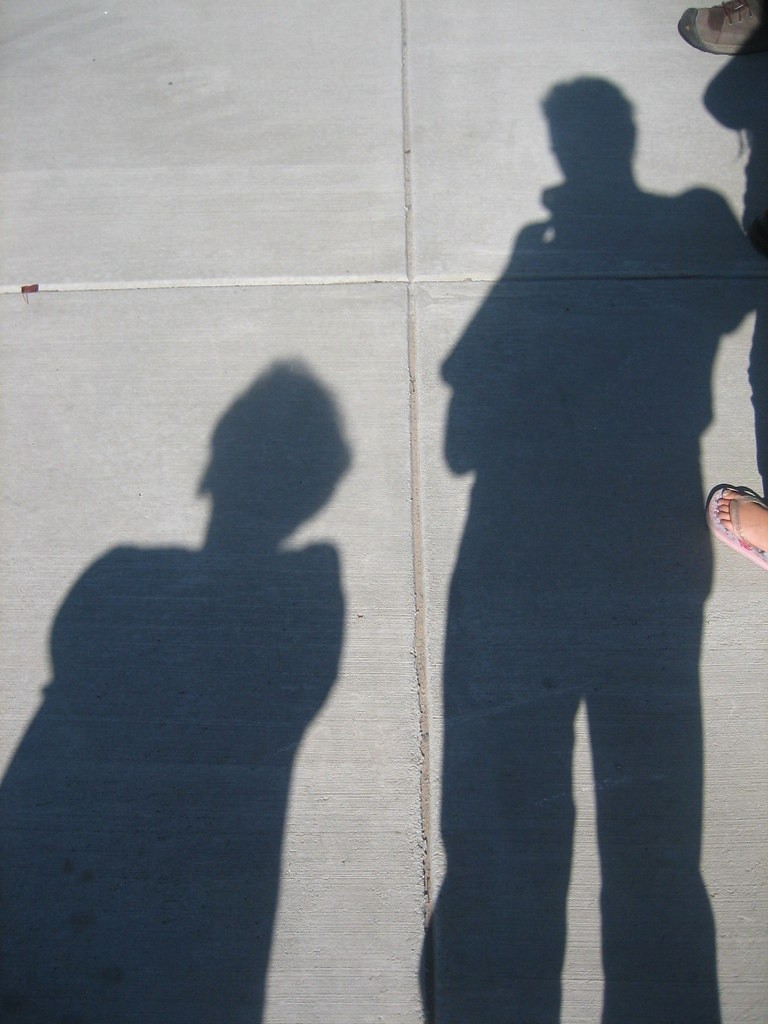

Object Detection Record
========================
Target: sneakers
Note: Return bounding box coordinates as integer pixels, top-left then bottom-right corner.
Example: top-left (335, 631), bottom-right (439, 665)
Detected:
top-left (678, 0), bottom-right (768, 55)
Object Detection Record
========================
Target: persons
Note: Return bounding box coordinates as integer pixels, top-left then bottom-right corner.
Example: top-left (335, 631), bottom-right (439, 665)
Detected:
top-left (706, 485), bottom-right (768, 572)
top-left (677, 0), bottom-right (768, 56)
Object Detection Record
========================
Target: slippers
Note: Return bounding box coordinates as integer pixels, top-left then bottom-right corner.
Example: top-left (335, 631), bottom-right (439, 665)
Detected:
top-left (704, 486), bottom-right (768, 572)
